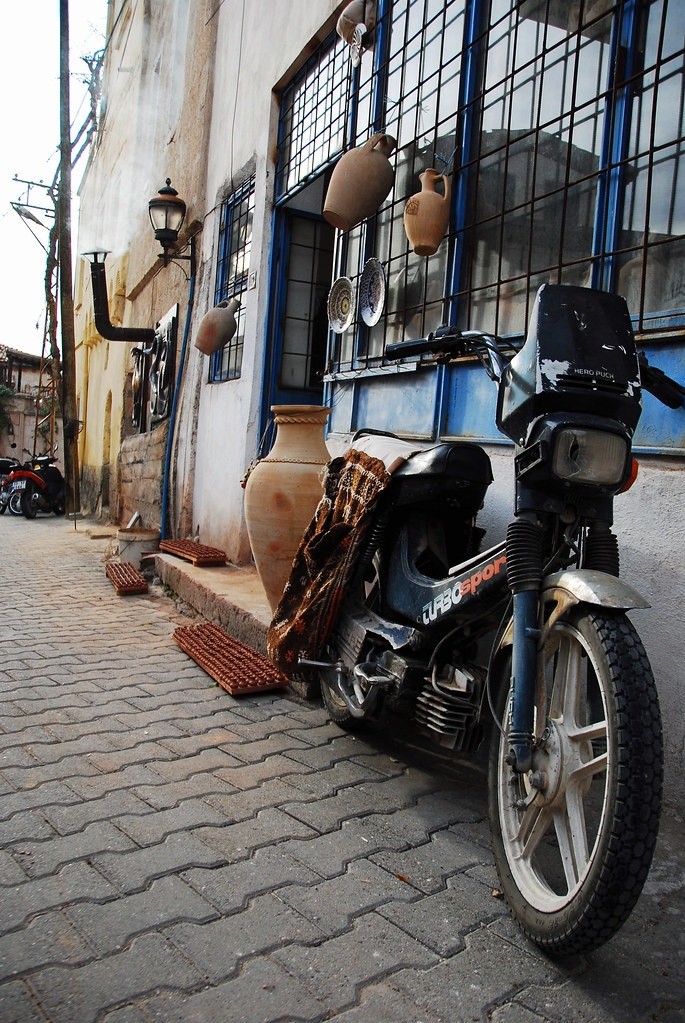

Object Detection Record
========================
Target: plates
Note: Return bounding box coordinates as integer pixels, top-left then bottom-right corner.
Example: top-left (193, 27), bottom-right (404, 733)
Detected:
top-left (327, 277), bottom-right (356, 334)
top-left (359, 258), bottom-right (386, 326)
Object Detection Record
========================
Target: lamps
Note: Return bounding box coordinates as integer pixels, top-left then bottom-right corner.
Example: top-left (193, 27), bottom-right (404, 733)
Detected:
top-left (148, 177), bottom-right (199, 281)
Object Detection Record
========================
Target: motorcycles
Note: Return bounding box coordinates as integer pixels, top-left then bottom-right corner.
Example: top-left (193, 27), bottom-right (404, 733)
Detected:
top-left (0, 455), bottom-right (22, 515)
top-left (297, 282), bottom-right (685, 965)
top-left (9, 445), bottom-right (67, 519)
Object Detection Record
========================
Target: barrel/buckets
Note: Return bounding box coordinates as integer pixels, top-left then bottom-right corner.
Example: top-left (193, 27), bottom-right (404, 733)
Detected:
top-left (115, 529), bottom-right (161, 570)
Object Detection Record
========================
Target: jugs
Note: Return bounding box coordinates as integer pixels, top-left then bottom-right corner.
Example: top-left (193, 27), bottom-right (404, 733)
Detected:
top-left (195, 299), bottom-right (241, 355)
top-left (403, 168), bottom-right (451, 256)
top-left (322, 133), bottom-right (397, 230)
